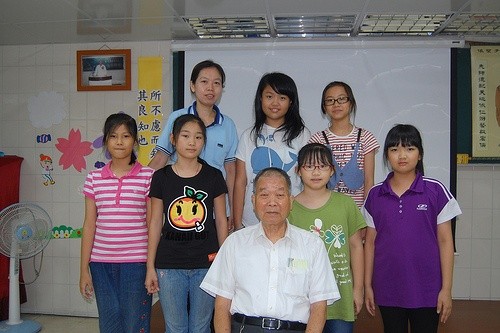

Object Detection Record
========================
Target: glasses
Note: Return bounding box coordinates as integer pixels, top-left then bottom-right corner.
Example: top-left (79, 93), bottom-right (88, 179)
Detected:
top-left (323, 97), bottom-right (351, 106)
top-left (301, 165), bottom-right (331, 170)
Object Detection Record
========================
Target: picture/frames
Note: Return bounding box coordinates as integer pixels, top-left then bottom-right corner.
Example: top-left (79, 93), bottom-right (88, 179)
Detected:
top-left (77, 48), bottom-right (131, 91)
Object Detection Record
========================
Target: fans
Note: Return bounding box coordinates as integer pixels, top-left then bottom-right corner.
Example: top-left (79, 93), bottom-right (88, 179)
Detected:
top-left (0, 201), bottom-right (53, 333)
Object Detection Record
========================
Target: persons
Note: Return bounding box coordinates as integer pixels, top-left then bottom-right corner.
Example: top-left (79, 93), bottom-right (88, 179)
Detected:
top-left (93, 60), bottom-right (108, 76)
top-left (287, 143), bottom-right (368, 333)
top-left (144, 114), bottom-right (229, 333)
top-left (307, 81), bottom-right (380, 246)
top-left (361, 124), bottom-right (462, 333)
top-left (232, 72), bottom-right (312, 231)
top-left (147, 60), bottom-right (240, 239)
top-left (79, 113), bottom-right (155, 333)
top-left (199, 167), bottom-right (341, 333)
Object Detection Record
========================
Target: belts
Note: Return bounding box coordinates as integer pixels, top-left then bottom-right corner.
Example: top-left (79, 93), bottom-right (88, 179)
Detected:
top-left (234, 312), bottom-right (307, 331)
